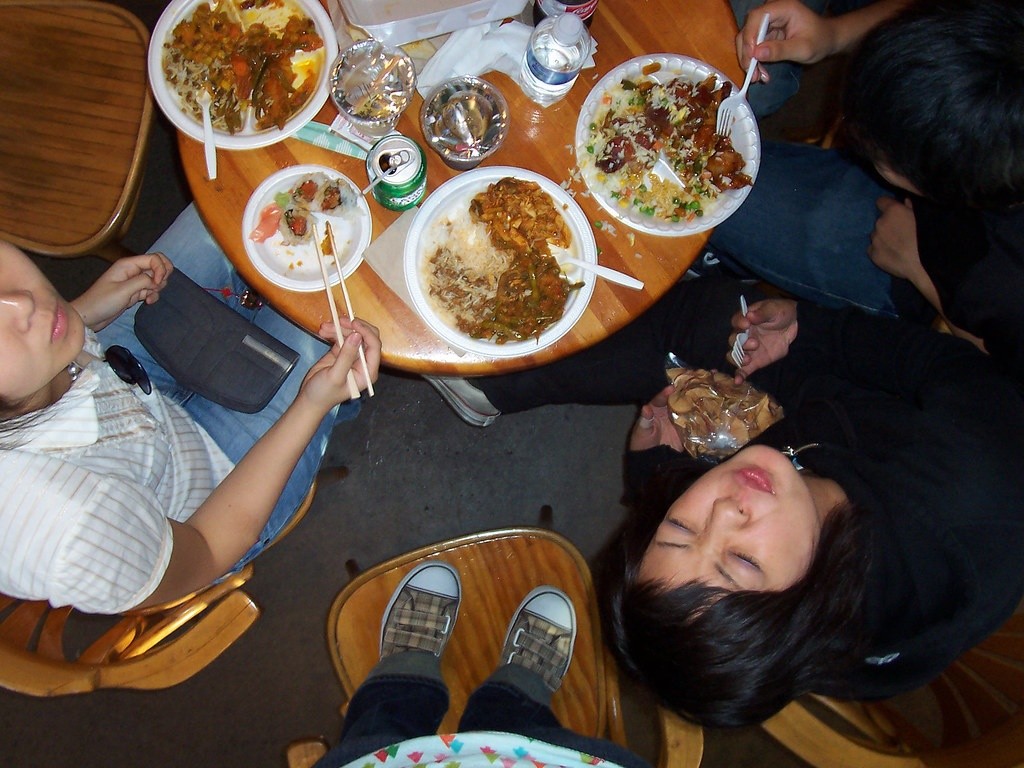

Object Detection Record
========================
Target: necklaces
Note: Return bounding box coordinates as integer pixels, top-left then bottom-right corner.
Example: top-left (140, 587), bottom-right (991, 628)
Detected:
top-left (67, 364), bottom-right (79, 381)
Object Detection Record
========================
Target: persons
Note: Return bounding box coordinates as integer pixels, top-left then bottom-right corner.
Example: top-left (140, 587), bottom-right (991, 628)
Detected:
top-left (0, 201), bottom-right (382, 614)
top-left (715, 0), bottom-right (1024, 355)
top-left (311, 561), bottom-right (652, 768)
top-left (420, 275), bottom-right (1024, 730)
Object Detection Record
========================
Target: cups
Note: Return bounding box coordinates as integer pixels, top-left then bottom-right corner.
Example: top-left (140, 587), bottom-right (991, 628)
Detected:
top-left (419, 75), bottom-right (511, 174)
top-left (327, 40), bottom-right (417, 138)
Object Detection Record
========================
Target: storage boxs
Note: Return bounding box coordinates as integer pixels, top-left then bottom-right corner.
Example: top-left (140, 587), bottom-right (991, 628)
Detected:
top-left (340, 0), bottom-right (529, 47)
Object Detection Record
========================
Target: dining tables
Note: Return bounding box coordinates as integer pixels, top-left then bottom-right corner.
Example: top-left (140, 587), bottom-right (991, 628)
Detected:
top-left (177, 0), bottom-right (749, 380)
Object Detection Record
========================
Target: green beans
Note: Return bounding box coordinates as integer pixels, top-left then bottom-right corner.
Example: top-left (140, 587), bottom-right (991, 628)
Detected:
top-left (587, 98), bottom-right (704, 258)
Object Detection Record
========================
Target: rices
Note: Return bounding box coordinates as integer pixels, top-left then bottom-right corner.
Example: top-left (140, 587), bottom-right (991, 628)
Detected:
top-left (161, 46), bottom-right (242, 130)
top-left (560, 80), bottom-right (722, 238)
top-left (422, 208), bottom-right (513, 329)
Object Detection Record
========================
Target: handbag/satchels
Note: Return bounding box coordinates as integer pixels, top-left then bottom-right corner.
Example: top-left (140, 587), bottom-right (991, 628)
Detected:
top-left (134, 268), bottom-right (301, 414)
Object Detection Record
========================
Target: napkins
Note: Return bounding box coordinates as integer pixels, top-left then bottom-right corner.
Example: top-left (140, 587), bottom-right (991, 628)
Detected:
top-left (415, 23), bottom-right (506, 100)
top-left (361, 206), bottom-right (467, 358)
top-left (489, 19), bottom-right (598, 85)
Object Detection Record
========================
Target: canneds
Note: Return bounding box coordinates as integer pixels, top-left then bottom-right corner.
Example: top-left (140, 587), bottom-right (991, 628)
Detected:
top-left (364, 135), bottom-right (427, 212)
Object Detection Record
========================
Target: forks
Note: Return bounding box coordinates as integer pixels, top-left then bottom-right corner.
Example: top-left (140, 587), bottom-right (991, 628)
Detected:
top-left (714, 10), bottom-right (771, 137)
top-left (731, 292), bottom-right (753, 370)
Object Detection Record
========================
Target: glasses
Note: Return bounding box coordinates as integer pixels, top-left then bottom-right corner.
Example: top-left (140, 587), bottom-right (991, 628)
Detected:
top-left (104, 345), bottom-right (151, 395)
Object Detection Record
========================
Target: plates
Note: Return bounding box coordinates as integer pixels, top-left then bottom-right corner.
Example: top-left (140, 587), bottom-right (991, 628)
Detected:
top-left (403, 164), bottom-right (600, 360)
top-left (575, 53), bottom-right (761, 235)
top-left (241, 163), bottom-right (373, 294)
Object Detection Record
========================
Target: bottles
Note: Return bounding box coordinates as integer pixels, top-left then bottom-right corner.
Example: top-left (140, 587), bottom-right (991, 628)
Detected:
top-left (517, 12), bottom-right (593, 108)
top-left (533, 0), bottom-right (600, 30)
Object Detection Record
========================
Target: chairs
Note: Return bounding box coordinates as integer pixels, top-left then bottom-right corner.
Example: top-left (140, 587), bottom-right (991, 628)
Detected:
top-left (0, 466), bottom-right (352, 698)
top-left (761, 646), bottom-right (1024, 768)
top-left (0, 0), bottom-right (156, 264)
top-left (284, 504), bottom-right (705, 768)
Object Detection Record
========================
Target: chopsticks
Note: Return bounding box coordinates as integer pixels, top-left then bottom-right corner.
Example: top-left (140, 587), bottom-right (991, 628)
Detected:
top-left (312, 220), bottom-right (376, 399)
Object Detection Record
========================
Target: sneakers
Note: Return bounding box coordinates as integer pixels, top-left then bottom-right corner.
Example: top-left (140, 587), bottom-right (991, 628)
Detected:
top-left (422, 374), bottom-right (501, 427)
top-left (380, 560), bottom-right (462, 662)
top-left (500, 585), bottom-right (577, 692)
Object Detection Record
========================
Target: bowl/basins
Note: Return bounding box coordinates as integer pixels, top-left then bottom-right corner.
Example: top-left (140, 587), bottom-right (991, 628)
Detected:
top-left (146, 0), bottom-right (340, 151)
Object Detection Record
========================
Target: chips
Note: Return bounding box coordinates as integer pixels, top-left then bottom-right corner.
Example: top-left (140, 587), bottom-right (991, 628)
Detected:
top-left (667, 366), bottom-right (777, 459)
